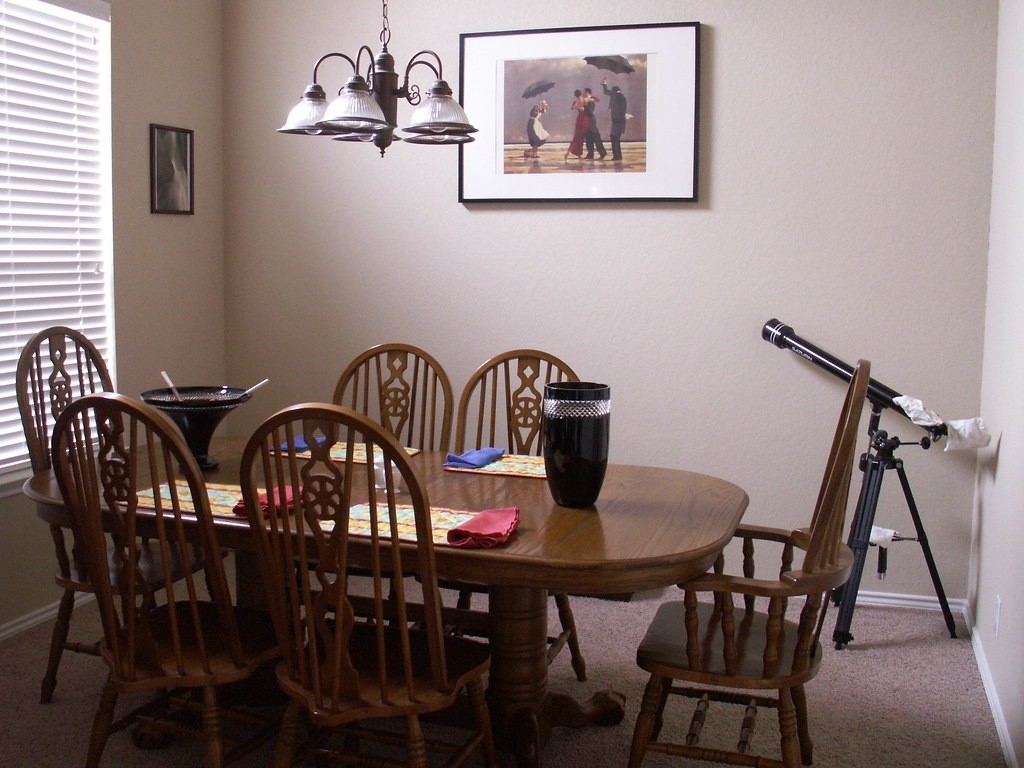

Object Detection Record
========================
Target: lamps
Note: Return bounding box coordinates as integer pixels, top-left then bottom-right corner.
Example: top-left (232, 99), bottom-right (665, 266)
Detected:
top-left (276, 0), bottom-right (479, 159)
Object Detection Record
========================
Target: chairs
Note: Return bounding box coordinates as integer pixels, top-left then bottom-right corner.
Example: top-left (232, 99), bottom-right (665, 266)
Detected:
top-left (407, 349), bottom-right (593, 687)
top-left (625, 358), bottom-right (873, 768)
top-left (50, 391), bottom-right (305, 768)
top-left (318, 342), bottom-right (456, 639)
top-left (238, 400), bottom-right (499, 768)
top-left (14, 327), bottom-right (229, 708)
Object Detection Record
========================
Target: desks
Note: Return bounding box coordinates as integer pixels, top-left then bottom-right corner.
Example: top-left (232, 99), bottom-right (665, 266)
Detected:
top-left (21, 431), bottom-right (752, 767)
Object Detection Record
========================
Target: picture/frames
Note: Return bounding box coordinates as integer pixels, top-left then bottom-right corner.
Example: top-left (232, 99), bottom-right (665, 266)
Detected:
top-left (149, 122), bottom-right (196, 216)
top-left (452, 22), bottom-right (700, 206)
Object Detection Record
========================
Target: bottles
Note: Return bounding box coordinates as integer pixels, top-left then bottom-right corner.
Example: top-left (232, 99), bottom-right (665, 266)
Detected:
top-left (373, 457), bottom-right (386, 488)
top-left (383, 463), bottom-right (402, 494)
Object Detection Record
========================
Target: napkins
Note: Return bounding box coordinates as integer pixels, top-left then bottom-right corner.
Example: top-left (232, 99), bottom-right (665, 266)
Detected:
top-left (268, 435), bottom-right (327, 453)
top-left (232, 484), bottom-right (304, 521)
top-left (446, 505), bottom-right (519, 549)
top-left (441, 448), bottom-right (506, 469)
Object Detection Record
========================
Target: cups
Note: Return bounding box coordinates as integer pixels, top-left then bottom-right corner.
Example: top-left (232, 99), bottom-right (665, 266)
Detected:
top-left (542, 381), bottom-right (612, 504)
